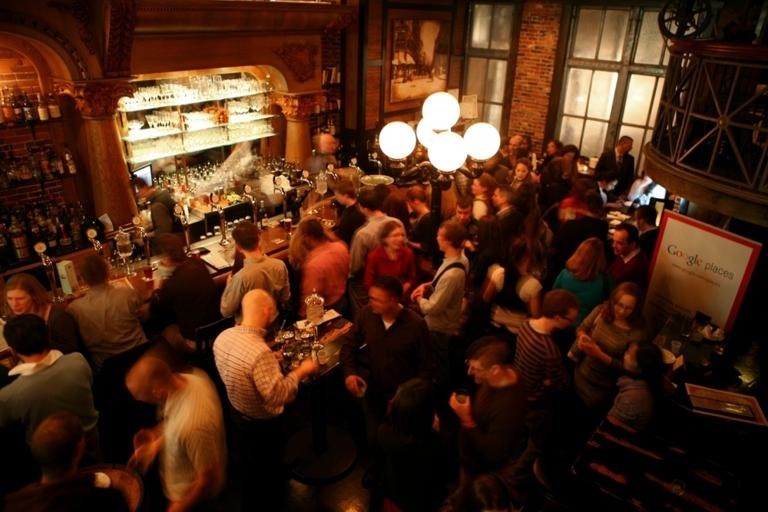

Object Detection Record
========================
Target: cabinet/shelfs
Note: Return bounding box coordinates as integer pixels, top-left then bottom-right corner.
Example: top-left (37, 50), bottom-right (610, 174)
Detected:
top-left (114, 79), bottom-right (281, 165)
top-left (0, 102), bottom-right (94, 271)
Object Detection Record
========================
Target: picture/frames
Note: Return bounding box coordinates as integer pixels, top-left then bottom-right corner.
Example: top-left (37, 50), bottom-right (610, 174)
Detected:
top-left (379, 2), bottom-right (457, 118)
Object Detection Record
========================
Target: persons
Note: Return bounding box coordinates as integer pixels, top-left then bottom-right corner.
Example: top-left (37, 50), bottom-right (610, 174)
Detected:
top-left (0, 137), bottom-right (664, 512)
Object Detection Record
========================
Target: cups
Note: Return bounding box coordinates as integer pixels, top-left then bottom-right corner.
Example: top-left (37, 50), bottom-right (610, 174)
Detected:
top-left (282, 329), bottom-right (326, 366)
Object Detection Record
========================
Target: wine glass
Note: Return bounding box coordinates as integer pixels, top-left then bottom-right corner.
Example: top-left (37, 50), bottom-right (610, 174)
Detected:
top-left (115, 239), bottom-right (136, 277)
top-left (123, 73), bottom-right (277, 162)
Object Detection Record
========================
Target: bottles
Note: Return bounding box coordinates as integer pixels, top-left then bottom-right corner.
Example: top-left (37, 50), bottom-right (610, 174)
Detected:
top-left (0, 144), bottom-right (77, 184)
top-left (0, 81), bottom-right (65, 125)
top-left (0, 197), bottom-right (93, 268)
top-left (154, 151), bottom-right (294, 201)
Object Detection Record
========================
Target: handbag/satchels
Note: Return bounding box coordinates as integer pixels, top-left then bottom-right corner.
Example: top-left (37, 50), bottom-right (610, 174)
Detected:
top-left (409, 284), bottom-right (434, 315)
top-left (568, 329), bottom-right (593, 363)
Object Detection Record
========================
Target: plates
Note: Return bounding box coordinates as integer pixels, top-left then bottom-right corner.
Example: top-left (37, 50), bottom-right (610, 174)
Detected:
top-left (360, 174), bottom-right (396, 188)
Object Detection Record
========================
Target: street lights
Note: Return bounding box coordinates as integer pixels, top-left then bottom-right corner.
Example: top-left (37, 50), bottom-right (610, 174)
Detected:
top-left (375, 91), bottom-right (502, 256)
